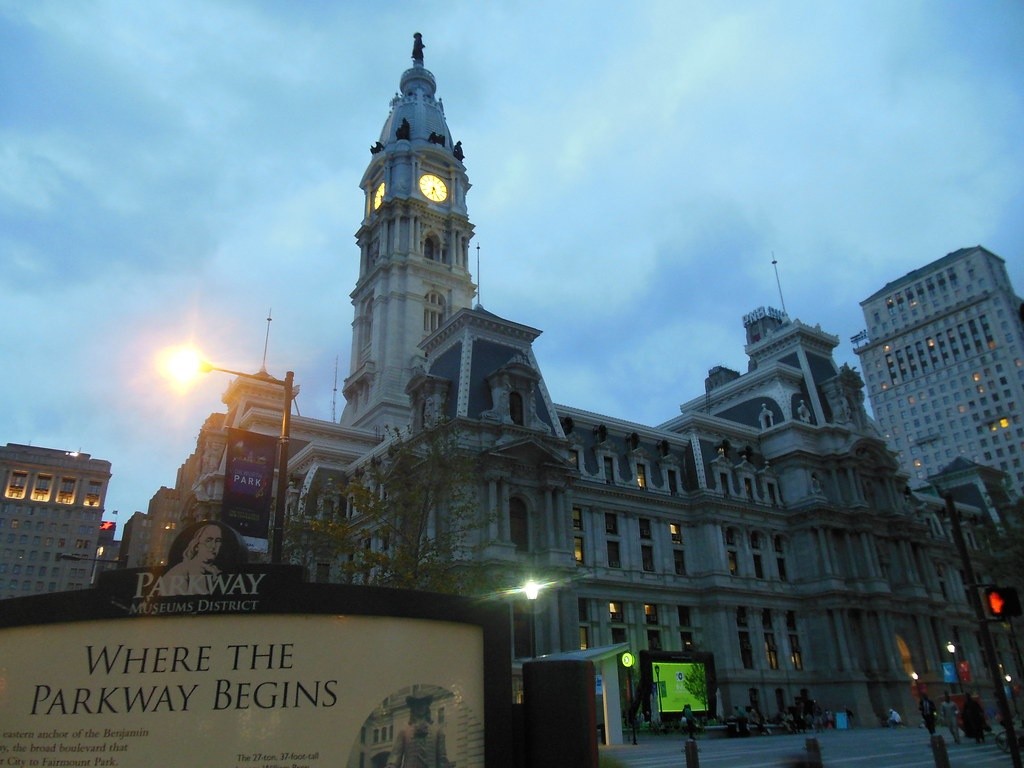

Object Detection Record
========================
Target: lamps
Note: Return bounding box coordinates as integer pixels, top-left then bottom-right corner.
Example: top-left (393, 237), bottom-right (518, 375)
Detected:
top-left (612, 614), bottom-right (622, 620)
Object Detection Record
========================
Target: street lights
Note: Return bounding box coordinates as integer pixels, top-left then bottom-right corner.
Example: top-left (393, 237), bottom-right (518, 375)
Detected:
top-left (911, 671), bottom-right (923, 702)
top-left (946, 641), bottom-right (972, 701)
top-left (172, 353), bottom-right (295, 560)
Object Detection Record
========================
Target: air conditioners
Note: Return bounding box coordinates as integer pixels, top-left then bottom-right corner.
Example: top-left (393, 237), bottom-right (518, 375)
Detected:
top-left (610, 562), bottom-right (619, 567)
top-left (677, 569), bottom-right (685, 575)
top-left (606, 524), bottom-right (613, 529)
top-left (640, 487), bottom-right (648, 491)
top-left (645, 565), bottom-right (653, 570)
top-left (673, 534), bottom-right (681, 540)
top-left (574, 522), bottom-right (580, 526)
top-left (769, 645), bottom-right (777, 651)
top-left (607, 481), bottom-right (615, 486)
top-left (642, 530), bottom-right (649, 536)
top-left (673, 492), bottom-right (680, 497)
top-left (744, 644), bottom-right (751, 650)
top-left (654, 643), bottom-right (661, 648)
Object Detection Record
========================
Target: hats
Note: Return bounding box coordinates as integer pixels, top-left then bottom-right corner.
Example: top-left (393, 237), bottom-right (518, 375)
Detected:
top-left (889, 709), bottom-right (893, 713)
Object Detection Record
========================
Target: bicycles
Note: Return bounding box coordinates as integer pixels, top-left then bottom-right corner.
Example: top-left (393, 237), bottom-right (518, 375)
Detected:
top-left (996, 717), bottom-right (1024, 752)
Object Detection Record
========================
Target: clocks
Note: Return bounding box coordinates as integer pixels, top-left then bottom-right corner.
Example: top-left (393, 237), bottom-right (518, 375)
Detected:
top-left (374, 183), bottom-right (385, 209)
top-left (419, 174), bottom-right (448, 202)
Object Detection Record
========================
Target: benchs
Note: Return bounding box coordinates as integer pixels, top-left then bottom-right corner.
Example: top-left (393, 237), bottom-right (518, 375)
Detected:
top-left (704, 724), bottom-right (797, 739)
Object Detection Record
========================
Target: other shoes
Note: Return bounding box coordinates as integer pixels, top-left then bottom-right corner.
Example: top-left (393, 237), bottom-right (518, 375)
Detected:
top-left (690, 737), bottom-right (695, 740)
top-left (954, 738), bottom-right (957, 742)
top-left (981, 737), bottom-right (985, 742)
top-left (957, 740), bottom-right (960, 744)
top-left (976, 739), bottom-right (981, 744)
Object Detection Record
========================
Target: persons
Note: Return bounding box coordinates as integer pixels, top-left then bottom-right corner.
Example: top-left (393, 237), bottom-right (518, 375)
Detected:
top-left (684, 704), bottom-right (698, 740)
top-left (734, 698), bottom-right (825, 736)
top-left (963, 692), bottom-right (986, 744)
top-left (940, 695), bottom-right (960, 746)
top-left (887, 708), bottom-right (902, 729)
top-left (844, 705), bottom-right (855, 729)
top-left (917, 693), bottom-right (937, 736)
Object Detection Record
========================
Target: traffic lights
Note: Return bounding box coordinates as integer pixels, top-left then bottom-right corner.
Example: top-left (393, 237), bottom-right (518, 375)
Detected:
top-left (985, 585), bottom-right (1021, 618)
